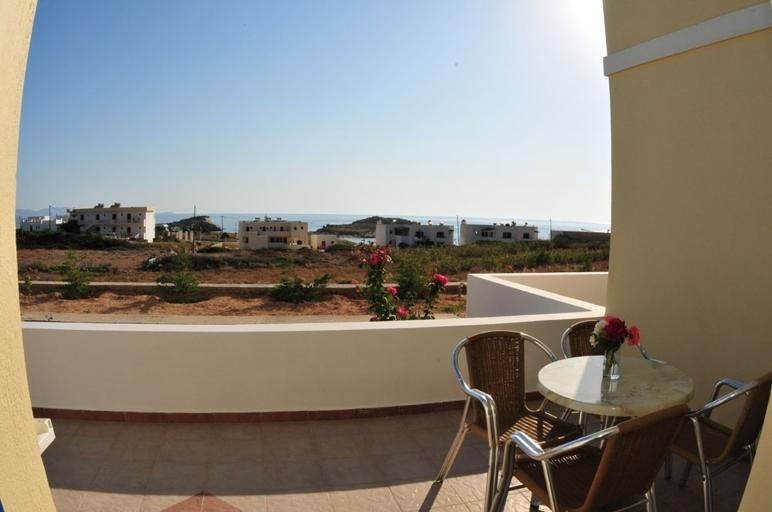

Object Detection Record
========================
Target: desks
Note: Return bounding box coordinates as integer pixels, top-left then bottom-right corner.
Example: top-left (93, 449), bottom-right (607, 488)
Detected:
top-left (535, 357), bottom-right (696, 445)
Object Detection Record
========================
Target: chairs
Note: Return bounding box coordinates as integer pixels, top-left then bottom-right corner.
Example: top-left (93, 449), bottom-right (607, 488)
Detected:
top-left (489, 402), bottom-right (689, 512)
top-left (435, 327), bottom-right (588, 512)
top-left (560, 318), bottom-right (666, 448)
top-left (660, 366), bottom-right (772, 512)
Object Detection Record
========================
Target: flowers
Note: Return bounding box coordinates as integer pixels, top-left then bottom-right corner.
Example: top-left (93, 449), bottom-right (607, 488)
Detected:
top-left (590, 314), bottom-right (639, 374)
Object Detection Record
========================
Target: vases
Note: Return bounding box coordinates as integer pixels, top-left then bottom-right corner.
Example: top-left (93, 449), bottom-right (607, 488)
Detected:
top-left (602, 348), bottom-right (621, 380)
